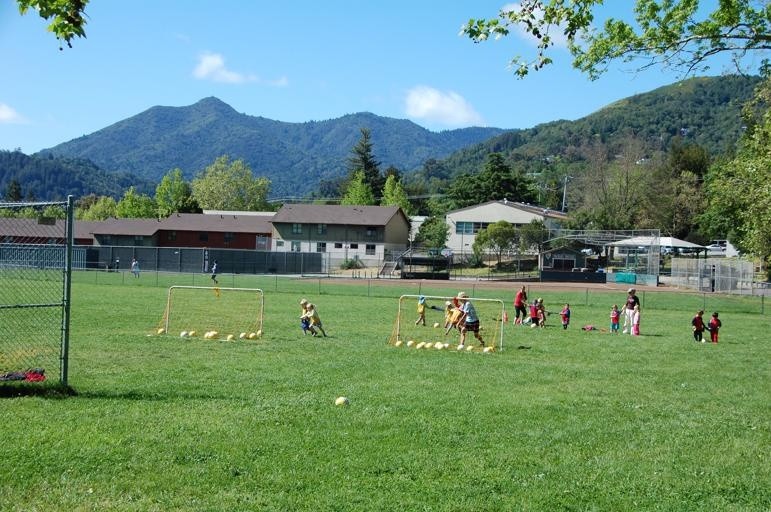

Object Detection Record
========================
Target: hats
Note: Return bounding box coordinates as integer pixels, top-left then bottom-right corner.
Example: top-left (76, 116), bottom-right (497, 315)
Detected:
top-left (419, 296), bottom-right (425, 304)
top-left (458, 292), bottom-right (468, 297)
top-left (627, 288), bottom-right (632, 292)
top-left (300, 299), bottom-right (307, 305)
top-left (446, 301), bottom-right (451, 306)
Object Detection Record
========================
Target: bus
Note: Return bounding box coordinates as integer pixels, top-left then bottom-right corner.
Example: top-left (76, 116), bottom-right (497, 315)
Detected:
top-left (699, 264), bottom-right (738, 291)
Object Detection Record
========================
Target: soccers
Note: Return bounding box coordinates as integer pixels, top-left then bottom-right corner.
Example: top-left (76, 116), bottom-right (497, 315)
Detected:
top-left (336, 397), bottom-right (349, 406)
top-left (467, 346), bottom-right (475, 352)
top-left (180, 332), bottom-right (188, 338)
top-left (408, 341), bottom-right (449, 350)
top-left (227, 335), bottom-right (234, 341)
top-left (483, 347), bottom-right (491, 353)
top-left (190, 331), bottom-right (197, 337)
top-left (531, 323), bottom-right (536, 328)
top-left (701, 337), bottom-right (708, 343)
top-left (457, 345), bottom-right (464, 351)
top-left (434, 323), bottom-right (439, 328)
top-left (205, 331), bottom-right (218, 338)
top-left (158, 328), bottom-right (166, 334)
top-left (396, 341), bottom-right (403, 347)
top-left (240, 330), bottom-right (263, 340)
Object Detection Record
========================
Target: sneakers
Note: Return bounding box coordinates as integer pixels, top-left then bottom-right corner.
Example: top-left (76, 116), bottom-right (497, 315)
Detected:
top-left (304, 332), bottom-right (327, 337)
top-left (480, 341), bottom-right (484, 346)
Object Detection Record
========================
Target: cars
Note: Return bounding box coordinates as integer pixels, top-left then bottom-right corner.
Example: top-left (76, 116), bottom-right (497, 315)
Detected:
top-left (580, 236), bottom-right (728, 256)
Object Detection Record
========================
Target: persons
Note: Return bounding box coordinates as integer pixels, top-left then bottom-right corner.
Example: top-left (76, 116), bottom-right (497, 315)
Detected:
top-left (301, 303), bottom-right (327, 338)
top-left (131, 258), bottom-right (141, 279)
top-left (692, 310), bottom-right (708, 342)
top-left (416, 296), bottom-right (433, 327)
top-left (211, 260), bottom-right (219, 284)
top-left (631, 305), bottom-right (641, 335)
top-left (444, 285), bottom-right (571, 347)
top-left (623, 287), bottom-right (641, 335)
top-left (610, 304), bottom-right (624, 335)
top-left (300, 297), bottom-right (314, 337)
top-left (707, 312), bottom-right (722, 344)
